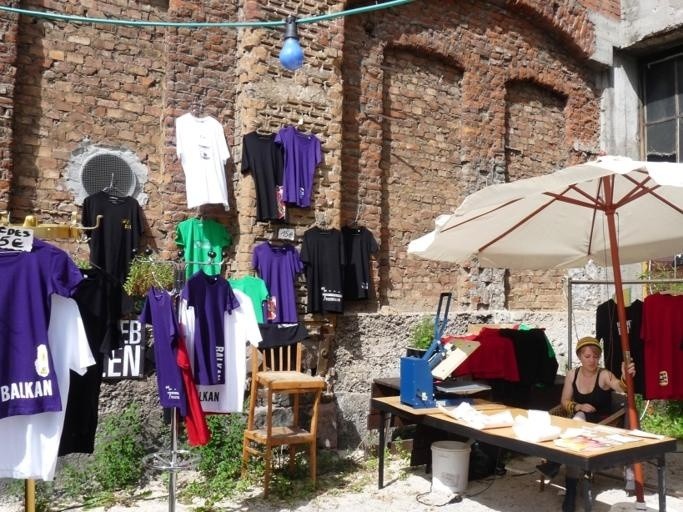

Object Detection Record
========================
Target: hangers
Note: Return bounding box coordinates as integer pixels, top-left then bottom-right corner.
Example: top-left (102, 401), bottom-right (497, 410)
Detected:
top-left (184, 102), bottom-right (315, 141)
top-left (197, 261), bottom-right (251, 284)
top-left (97, 178), bottom-right (128, 201)
top-left (61, 237), bottom-right (101, 273)
top-left (313, 212), bottom-right (367, 236)
top-left (264, 224), bottom-right (291, 253)
top-left (654, 279), bottom-right (683, 298)
top-left (147, 268), bottom-right (168, 299)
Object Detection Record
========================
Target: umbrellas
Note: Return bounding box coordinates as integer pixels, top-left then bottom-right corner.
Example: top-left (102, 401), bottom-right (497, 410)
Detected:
top-left (405, 154), bottom-right (682, 506)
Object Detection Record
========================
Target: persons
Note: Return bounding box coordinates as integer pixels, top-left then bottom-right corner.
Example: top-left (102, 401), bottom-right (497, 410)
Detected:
top-left (533, 335), bottom-right (636, 512)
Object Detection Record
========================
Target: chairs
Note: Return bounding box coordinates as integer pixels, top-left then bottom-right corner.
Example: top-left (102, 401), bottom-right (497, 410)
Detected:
top-left (237, 321), bottom-right (325, 499)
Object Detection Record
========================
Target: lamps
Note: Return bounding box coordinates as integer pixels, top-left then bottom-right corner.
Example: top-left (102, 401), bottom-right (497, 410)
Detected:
top-left (277, 14), bottom-right (306, 71)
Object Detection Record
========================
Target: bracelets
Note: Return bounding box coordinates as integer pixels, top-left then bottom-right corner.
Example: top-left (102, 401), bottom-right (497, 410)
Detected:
top-left (618, 378), bottom-right (627, 393)
top-left (564, 402), bottom-right (575, 414)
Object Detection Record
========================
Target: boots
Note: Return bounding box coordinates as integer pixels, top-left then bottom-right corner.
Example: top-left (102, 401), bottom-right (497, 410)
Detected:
top-left (535, 460), bottom-right (562, 480)
top-left (562, 473), bottom-right (580, 512)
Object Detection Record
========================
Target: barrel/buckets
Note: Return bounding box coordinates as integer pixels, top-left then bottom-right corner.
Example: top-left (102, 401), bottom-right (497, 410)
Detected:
top-left (429, 441), bottom-right (470, 494)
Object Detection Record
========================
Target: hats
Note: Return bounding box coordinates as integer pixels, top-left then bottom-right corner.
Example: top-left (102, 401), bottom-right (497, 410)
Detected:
top-left (576, 337), bottom-right (602, 352)
